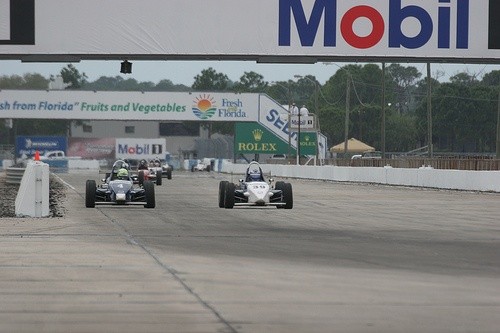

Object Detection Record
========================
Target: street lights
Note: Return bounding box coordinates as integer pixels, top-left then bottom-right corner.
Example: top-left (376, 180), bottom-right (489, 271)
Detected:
top-left (291, 99), bottom-right (309, 165)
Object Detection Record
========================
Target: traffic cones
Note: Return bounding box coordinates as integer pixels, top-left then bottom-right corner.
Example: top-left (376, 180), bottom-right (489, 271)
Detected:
top-left (34, 149), bottom-right (40, 160)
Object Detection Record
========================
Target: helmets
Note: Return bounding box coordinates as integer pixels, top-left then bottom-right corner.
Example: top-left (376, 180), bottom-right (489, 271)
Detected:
top-left (117, 169), bottom-right (129, 180)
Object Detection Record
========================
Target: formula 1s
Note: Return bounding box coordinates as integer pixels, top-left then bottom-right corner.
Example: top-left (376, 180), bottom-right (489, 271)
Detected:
top-left (85, 158), bottom-right (174, 210)
top-left (217, 160), bottom-right (293, 210)
top-left (191, 162), bottom-right (210, 172)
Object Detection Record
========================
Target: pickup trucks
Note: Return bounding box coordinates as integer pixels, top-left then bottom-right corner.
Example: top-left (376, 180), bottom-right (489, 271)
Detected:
top-left (41, 152), bottom-right (82, 160)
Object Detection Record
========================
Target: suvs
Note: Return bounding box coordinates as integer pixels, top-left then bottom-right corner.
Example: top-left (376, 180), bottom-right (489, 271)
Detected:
top-left (351, 151), bottom-right (382, 159)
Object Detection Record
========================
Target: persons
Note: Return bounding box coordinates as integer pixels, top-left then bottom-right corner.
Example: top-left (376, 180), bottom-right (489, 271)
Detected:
top-left (247, 164), bottom-right (262, 182)
top-left (300, 105), bottom-right (310, 116)
top-left (289, 103), bottom-right (299, 116)
top-left (117, 159), bottom-right (159, 180)
